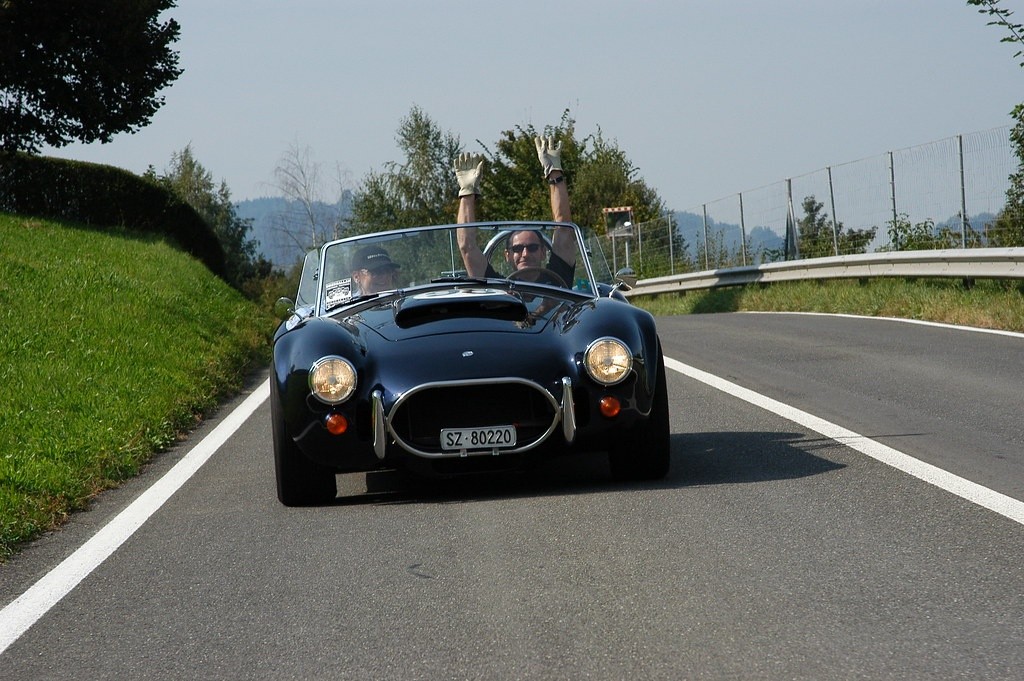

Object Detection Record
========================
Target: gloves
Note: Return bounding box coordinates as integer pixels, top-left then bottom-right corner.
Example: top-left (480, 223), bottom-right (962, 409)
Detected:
top-left (534, 134), bottom-right (565, 179)
top-left (453, 152), bottom-right (484, 199)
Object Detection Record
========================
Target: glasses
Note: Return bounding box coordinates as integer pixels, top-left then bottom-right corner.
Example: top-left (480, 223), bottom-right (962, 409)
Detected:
top-left (507, 243), bottom-right (543, 252)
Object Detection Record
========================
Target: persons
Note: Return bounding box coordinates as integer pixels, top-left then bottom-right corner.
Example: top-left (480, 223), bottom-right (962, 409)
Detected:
top-left (454, 135), bottom-right (577, 293)
top-left (350, 246), bottom-right (393, 297)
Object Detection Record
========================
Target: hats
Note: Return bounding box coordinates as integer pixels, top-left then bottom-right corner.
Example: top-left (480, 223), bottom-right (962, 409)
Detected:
top-left (354, 246), bottom-right (402, 270)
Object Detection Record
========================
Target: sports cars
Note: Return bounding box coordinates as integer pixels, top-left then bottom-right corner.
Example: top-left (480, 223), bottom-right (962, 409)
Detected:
top-left (266, 220), bottom-right (672, 510)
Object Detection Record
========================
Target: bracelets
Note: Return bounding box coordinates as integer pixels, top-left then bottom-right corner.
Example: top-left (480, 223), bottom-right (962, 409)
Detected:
top-left (548, 176), bottom-right (564, 184)
